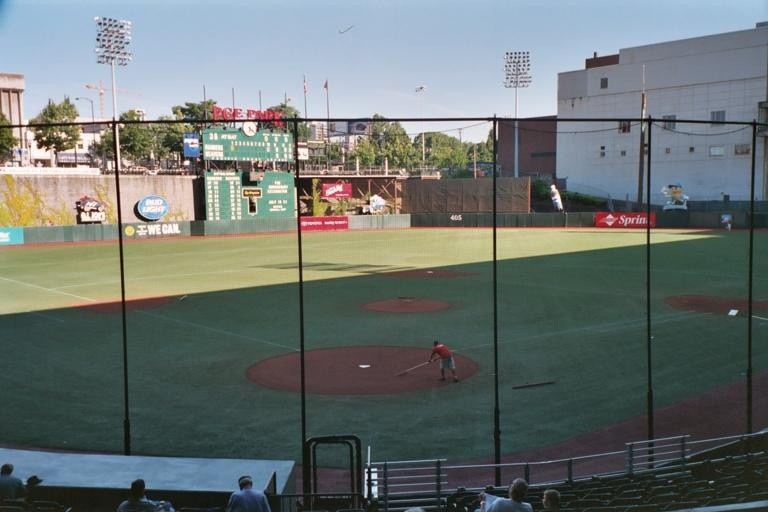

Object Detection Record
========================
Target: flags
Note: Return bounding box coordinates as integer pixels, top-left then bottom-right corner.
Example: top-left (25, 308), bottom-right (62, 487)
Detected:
top-left (323, 80), bottom-right (328, 89)
top-left (303, 79), bottom-right (307, 92)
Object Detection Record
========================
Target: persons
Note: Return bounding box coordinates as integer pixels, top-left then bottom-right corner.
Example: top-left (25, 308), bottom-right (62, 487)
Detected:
top-left (429, 340), bottom-right (458, 382)
top-left (471, 484), bottom-right (496, 506)
top-left (23, 472), bottom-right (44, 499)
top-left (479, 477), bottom-right (533, 512)
top-left (542, 489), bottom-right (563, 507)
top-left (117, 478), bottom-right (155, 510)
top-left (725, 220), bottom-right (732, 231)
top-left (225, 474), bottom-right (272, 512)
top-left (447, 486), bottom-right (466, 503)
top-left (0, 462), bottom-right (27, 499)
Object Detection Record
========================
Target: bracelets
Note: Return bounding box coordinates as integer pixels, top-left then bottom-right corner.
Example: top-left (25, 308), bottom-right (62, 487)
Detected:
top-left (480, 500), bottom-right (486, 504)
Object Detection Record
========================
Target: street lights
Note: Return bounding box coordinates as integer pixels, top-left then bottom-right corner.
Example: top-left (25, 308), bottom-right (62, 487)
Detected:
top-left (75, 96), bottom-right (94, 120)
top-left (503, 50), bottom-right (531, 176)
top-left (93, 16), bottom-right (132, 173)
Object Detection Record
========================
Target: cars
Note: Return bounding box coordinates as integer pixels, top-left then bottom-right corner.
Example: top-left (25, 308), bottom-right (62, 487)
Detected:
top-left (128, 166), bottom-right (150, 175)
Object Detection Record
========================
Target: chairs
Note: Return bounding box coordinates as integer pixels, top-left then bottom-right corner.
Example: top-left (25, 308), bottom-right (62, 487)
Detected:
top-left (445, 486), bottom-right (616, 512)
top-left (605, 455), bottom-right (768, 511)
top-left (0, 490), bottom-right (69, 512)
